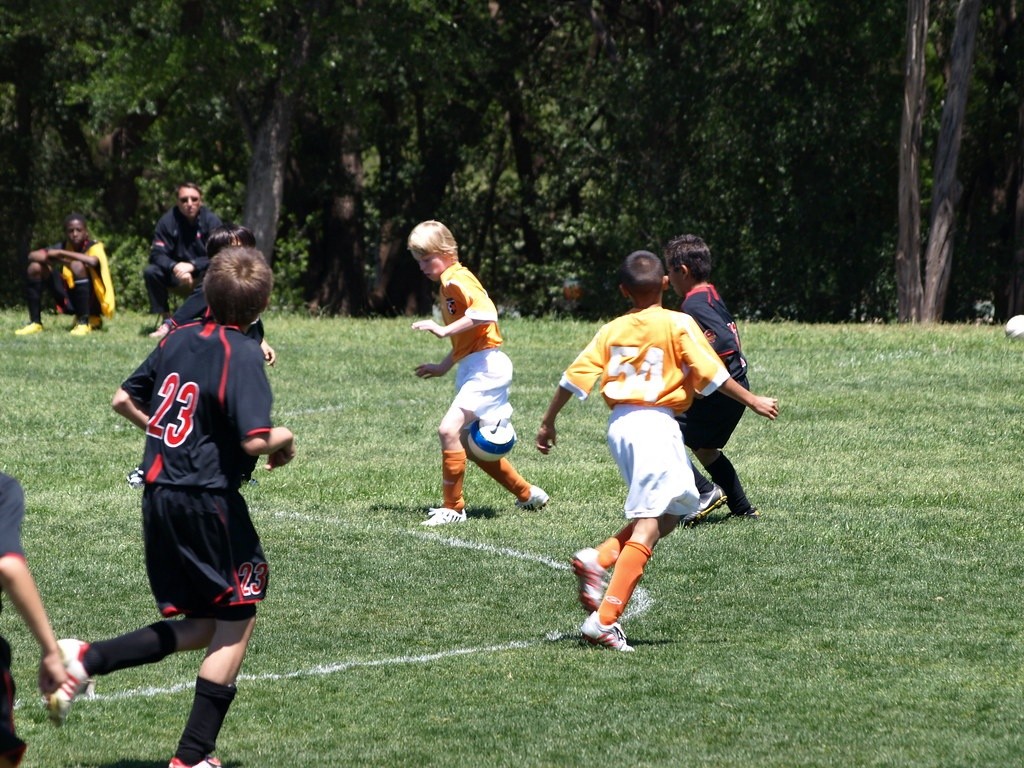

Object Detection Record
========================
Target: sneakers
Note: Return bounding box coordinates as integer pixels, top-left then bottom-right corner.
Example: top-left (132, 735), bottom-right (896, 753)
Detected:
top-left (70, 321), bottom-right (93, 337)
top-left (169, 755), bottom-right (223, 768)
top-left (572, 546), bottom-right (612, 611)
top-left (421, 504), bottom-right (466, 526)
top-left (580, 610), bottom-right (635, 653)
top-left (722, 504), bottom-right (760, 522)
top-left (680, 482), bottom-right (728, 526)
top-left (15, 320), bottom-right (44, 335)
top-left (515, 484), bottom-right (549, 511)
top-left (49, 639), bottom-right (98, 728)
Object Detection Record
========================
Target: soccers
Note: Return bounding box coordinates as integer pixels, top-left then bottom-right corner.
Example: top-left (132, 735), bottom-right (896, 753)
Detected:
top-left (468, 418), bottom-right (514, 462)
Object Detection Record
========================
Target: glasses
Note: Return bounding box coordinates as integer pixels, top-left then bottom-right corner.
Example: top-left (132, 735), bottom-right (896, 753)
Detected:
top-left (178, 196), bottom-right (201, 205)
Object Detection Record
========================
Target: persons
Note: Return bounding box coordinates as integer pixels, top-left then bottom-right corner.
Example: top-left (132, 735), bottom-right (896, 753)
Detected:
top-left (43, 242), bottom-right (297, 768)
top-left (142, 183), bottom-right (225, 328)
top-left (662, 233), bottom-right (760, 525)
top-left (148, 223), bottom-right (276, 367)
top-left (534, 248), bottom-right (778, 652)
top-left (408, 218), bottom-right (549, 526)
top-left (14, 213), bottom-right (115, 337)
top-left (0, 470), bottom-right (67, 768)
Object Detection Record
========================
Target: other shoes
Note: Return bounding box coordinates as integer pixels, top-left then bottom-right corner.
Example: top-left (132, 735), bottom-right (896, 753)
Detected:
top-left (127, 467), bottom-right (143, 490)
top-left (150, 323), bottom-right (172, 338)
